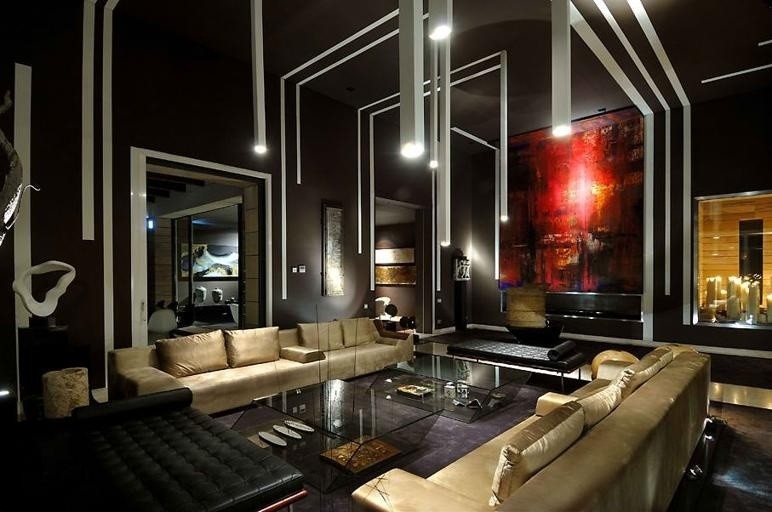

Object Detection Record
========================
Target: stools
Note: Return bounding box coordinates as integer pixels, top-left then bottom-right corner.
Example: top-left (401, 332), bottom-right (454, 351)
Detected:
top-left (42, 367), bottom-right (89, 418)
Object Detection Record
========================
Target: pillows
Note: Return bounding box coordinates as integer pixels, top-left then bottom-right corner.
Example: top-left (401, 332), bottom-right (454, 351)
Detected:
top-left (299, 321), bottom-right (344, 351)
top-left (341, 317), bottom-right (376, 348)
top-left (609, 346), bottom-right (673, 399)
top-left (155, 328), bottom-right (228, 378)
top-left (576, 385), bottom-right (622, 430)
top-left (489, 400), bottom-right (585, 509)
top-left (223, 326), bottom-right (280, 368)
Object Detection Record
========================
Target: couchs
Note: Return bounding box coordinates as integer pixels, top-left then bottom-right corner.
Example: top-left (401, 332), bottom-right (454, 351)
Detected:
top-left (348, 347), bottom-right (712, 512)
top-left (447, 335), bottom-right (589, 394)
top-left (71, 387), bottom-right (309, 512)
top-left (107, 320), bottom-right (416, 415)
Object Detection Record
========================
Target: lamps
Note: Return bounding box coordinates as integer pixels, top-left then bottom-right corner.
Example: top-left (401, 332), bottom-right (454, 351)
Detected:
top-left (250, 0), bottom-right (267, 153)
top-left (429, 44), bottom-right (436, 167)
top-left (551, 0), bottom-right (572, 140)
top-left (500, 48), bottom-right (508, 224)
top-left (436, 42), bottom-right (451, 248)
top-left (429, 1), bottom-right (453, 45)
top-left (399, 0), bottom-right (426, 160)
